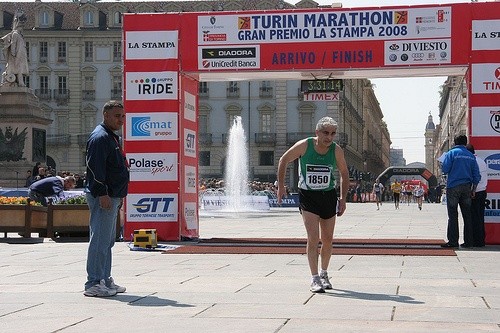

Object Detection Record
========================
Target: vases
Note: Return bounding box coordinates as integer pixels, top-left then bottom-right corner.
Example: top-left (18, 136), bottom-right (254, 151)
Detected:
top-left (0, 198), bottom-right (48, 238)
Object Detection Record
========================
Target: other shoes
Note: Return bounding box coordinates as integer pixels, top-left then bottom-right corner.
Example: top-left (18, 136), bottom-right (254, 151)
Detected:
top-left (474, 241), bottom-right (486, 247)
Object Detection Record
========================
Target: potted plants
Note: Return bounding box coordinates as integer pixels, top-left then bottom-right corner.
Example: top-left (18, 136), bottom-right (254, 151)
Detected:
top-left (47, 198), bottom-right (89, 237)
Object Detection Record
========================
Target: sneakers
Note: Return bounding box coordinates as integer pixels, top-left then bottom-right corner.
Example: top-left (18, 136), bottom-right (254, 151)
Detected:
top-left (441, 241), bottom-right (460, 249)
top-left (309, 279), bottom-right (325, 293)
top-left (105, 276), bottom-right (126, 293)
top-left (83, 279), bottom-right (117, 297)
top-left (319, 274), bottom-right (333, 290)
top-left (460, 242), bottom-right (473, 248)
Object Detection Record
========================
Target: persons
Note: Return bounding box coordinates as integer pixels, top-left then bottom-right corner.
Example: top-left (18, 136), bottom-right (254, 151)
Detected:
top-left (437, 134), bottom-right (488, 248)
top-left (277, 117), bottom-right (349, 292)
top-left (82, 101), bottom-right (129, 297)
top-left (335, 178), bottom-right (425, 210)
top-left (23, 162), bottom-right (87, 207)
top-left (6, 22), bottom-right (29, 87)
top-left (198, 177), bottom-right (291, 195)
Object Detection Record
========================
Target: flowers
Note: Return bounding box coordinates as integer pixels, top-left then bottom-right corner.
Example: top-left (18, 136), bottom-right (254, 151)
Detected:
top-left (0, 195), bottom-right (43, 207)
